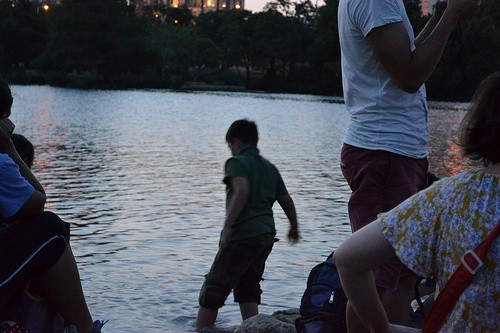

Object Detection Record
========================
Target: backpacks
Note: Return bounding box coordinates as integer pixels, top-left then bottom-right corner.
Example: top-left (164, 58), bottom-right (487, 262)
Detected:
top-left (295, 250), bottom-right (348, 332)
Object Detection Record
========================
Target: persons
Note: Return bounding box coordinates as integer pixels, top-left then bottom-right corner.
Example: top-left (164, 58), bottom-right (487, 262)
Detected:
top-left (332, 70), bottom-right (500, 333)
top-left (339, 1), bottom-right (474, 332)
top-left (0, 76), bottom-right (109, 333)
top-left (194, 119), bottom-right (299, 327)
